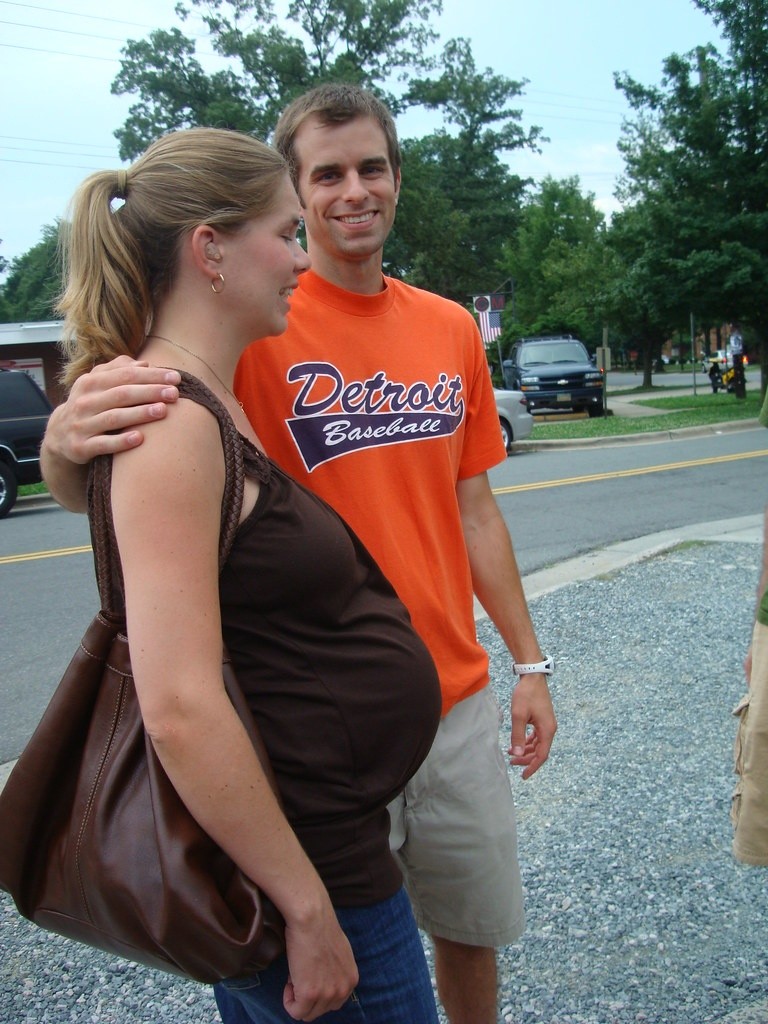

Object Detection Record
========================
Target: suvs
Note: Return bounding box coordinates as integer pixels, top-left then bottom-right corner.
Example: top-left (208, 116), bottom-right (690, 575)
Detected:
top-left (502, 332), bottom-right (604, 418)
top-left (0, 372), bottom-right (56, 519)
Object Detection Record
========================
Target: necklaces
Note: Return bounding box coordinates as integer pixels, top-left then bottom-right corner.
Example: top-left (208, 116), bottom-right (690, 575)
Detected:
top-left (147, 335), bottom-right (245, 414)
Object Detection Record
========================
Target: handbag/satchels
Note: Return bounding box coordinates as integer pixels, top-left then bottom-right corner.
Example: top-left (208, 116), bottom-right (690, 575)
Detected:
top-left (0, 366), bottom-right (287, 984)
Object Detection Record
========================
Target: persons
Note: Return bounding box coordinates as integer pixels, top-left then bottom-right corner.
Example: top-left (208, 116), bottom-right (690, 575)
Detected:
top-left (727, 381), bottom-right (768, 871)
top-left (40, 128), bottom-right (442, 1023)
top-left (41, 80), bottom-right (556, 1024)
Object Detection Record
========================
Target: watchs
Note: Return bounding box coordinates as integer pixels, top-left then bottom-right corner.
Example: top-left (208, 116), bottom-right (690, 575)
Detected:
top-left (511, 656), bottom-right (556, 676)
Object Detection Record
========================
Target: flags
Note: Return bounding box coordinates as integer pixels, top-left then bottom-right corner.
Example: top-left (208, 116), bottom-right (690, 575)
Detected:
top-left (479, 311), bottom-right (501, 350)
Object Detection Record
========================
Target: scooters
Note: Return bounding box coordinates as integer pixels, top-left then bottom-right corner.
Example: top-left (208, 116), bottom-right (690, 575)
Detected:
top-left (704, 356), bottom-right (748, 393)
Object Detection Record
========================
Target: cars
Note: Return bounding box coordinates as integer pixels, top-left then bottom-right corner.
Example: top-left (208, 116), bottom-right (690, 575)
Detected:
top-left (489, 383), bottom-right (529, 453)
top-left (701, 351), bottom-right (748, 375)
